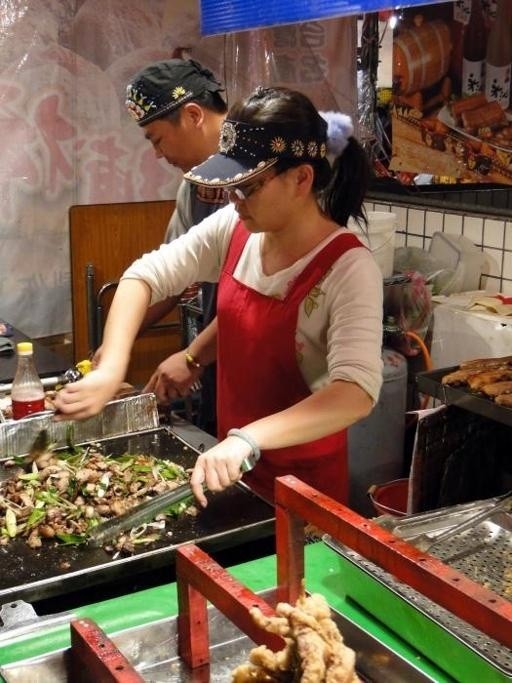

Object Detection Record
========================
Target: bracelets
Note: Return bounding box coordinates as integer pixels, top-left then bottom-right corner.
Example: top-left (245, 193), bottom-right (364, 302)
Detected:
top-left (185, 346), bottom-right (210, 370)
top-left (226, 427), bottom-right (262, 465)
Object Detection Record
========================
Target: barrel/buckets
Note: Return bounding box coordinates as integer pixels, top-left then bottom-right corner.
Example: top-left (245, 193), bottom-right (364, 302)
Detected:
top-left (366, 475), bottom-right (409, 515)
top-left (393, 17), bottom-right (454, 95)
top-left (343, 210), bottom-right (397, 280)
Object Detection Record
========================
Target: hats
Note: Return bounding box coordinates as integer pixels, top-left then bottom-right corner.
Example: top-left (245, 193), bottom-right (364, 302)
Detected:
top-left (124, 57), bottom-right (209, 127)
top-left (181, 85), bottom-right (330, 190)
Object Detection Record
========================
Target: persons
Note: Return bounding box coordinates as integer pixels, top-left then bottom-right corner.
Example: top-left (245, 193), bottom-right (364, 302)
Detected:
top-left (125, 56), bottom-right (237, 438)
top-left (50, 83), bottom-right (384, 508)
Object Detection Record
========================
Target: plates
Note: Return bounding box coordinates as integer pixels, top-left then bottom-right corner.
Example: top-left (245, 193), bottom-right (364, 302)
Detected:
top-left (437, 99), bottom-right (512, 153)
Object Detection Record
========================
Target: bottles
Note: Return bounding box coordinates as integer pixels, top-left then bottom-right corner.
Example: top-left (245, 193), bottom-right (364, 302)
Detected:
top-left (450, 1), bottom-right (511, 110)
top-left (10, 342), bottom-right (47, 420)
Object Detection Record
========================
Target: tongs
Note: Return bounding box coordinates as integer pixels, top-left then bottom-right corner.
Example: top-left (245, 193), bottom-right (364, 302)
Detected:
top-left (23, 368), bottom-right (84, 466)
top-left (85, 457), bottom-right (255, 549)
top-left (379, 494), bottom-right (512, 554)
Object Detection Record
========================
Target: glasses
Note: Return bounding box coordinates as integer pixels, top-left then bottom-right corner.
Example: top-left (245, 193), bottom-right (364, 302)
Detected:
top-left (222, 165), bottom-right (290, 200)
